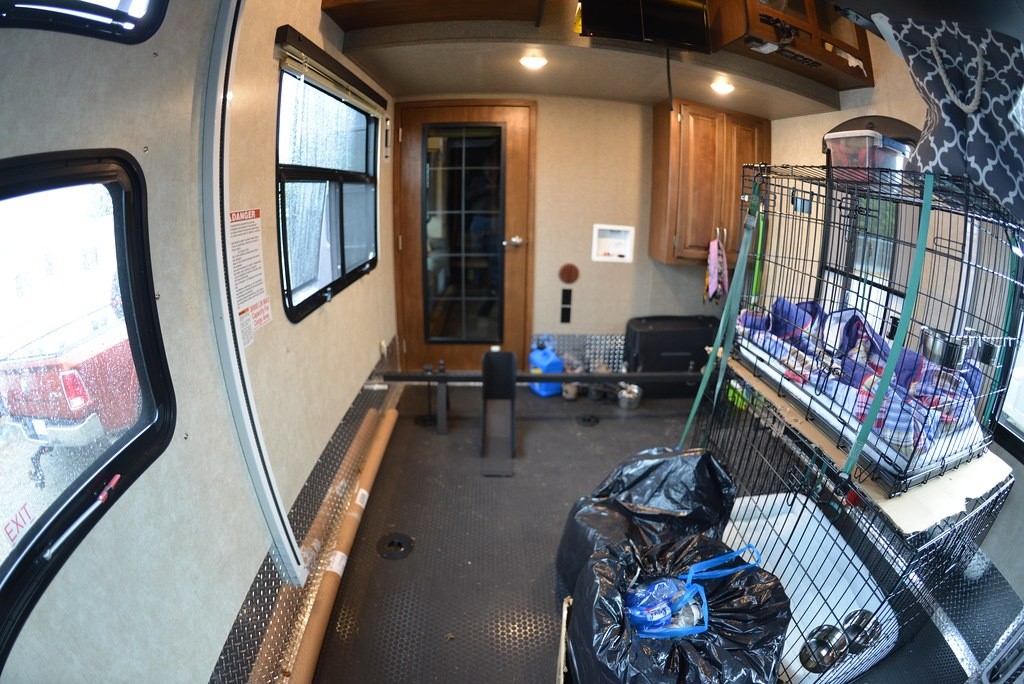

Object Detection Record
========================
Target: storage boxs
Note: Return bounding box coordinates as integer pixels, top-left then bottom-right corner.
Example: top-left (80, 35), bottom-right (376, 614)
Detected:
top-left (623, 314), bottom-right (723, 402)
top-left (825, 130), bottom-right (911, 195)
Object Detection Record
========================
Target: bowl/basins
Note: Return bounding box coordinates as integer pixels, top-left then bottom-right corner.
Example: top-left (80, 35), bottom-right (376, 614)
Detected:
top-left (800, 625), bottom-right (849, 673)
top-left (961, 328), bottom-right (1001, 366)
top-left (920, 325), bottom-right (968, 374)
top-left (843, 609), bottom-right (882, 655)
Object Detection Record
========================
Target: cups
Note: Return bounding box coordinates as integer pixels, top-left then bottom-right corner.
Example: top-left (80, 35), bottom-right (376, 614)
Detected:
top-left (617, 385), bottom-right (642, 409)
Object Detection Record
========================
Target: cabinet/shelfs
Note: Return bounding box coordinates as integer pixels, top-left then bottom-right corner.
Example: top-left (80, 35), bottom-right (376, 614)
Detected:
top-left (646, 97), bottom-right (775, 273)
top-left (707, 0), bottom-right (875, 91)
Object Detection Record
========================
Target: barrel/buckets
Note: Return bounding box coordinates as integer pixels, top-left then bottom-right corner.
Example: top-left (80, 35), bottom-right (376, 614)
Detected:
top-left (528, 344), bottom-right (564, 397)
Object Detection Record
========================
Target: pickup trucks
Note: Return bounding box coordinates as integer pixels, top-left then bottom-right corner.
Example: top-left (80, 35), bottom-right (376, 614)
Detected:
top-left (0, 296), bottom-right (140, 490)
